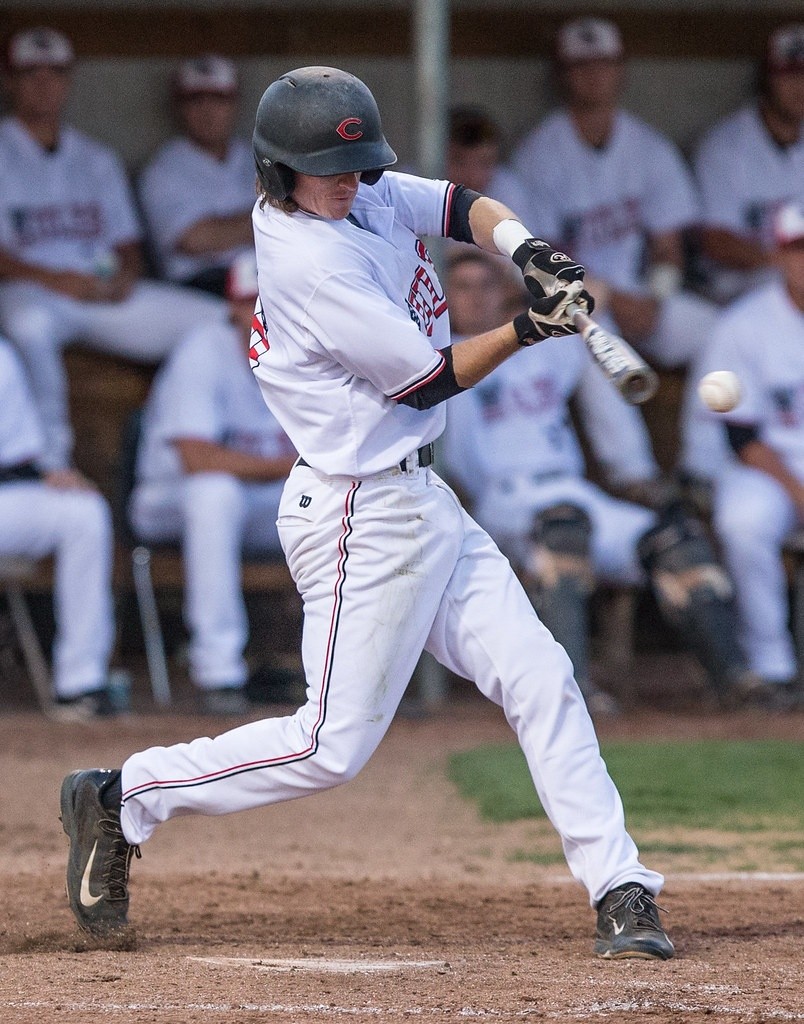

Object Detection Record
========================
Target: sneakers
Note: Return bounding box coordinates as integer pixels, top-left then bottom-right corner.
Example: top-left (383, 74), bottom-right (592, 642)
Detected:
top-left (60, 768), bottom-right (141, 946)
top-left (594, 883), bottom-right (674, 962)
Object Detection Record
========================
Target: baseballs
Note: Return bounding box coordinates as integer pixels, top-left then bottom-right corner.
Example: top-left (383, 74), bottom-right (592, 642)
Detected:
top-left (698, 369), bottom-right (743, 413)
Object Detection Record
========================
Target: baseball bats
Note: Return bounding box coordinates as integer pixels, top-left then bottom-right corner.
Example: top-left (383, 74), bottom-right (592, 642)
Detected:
top-left (566, 302), bottom-right (662, 407)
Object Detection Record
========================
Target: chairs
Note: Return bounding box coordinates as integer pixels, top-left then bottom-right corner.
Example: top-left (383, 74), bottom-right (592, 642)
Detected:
top-left (5, 409), bottom-right (293, 720)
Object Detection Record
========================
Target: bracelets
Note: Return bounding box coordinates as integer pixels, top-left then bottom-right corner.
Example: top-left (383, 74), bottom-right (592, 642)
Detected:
top-left (492, 219), bottom-right (534, 258)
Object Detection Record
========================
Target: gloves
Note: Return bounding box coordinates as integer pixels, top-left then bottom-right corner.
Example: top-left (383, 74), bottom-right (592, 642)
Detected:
top-left (513, 239), bottom-right (585, 299)
top-left (514, 280), bottom-right (594, 346)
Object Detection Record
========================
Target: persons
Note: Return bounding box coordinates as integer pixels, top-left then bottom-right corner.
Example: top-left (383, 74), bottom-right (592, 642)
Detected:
top-left (511, 16), bottom-right (719, 513)
top-left (0, 337), bottom-right (119, 721)
top-left (125, 248), bottom-right (298, 713)
top-left (692, 23), bottom-right (803, 301)
top-left (138, 52), bottom-right (258, 298)
top-left (674, 197), bottom-right (803, 709)
top-left (61, 66), bottom-right (676, 958)
top-left (428, 257), bottom-right (771, 705)
top-left (0, 28), bottom-right (233, 467)
top-left (452, 102), bottom-right (534, 243)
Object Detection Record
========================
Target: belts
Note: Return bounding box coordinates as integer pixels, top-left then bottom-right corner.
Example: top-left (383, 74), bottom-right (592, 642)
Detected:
top-left (295, 441), bottom-right (434, 472)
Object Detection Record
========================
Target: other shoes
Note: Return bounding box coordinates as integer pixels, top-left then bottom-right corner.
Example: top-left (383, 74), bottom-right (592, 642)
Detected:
top-left (53, 691), bottom-right (131, 723)
top-left (202, 686), bottom-right (248, 717)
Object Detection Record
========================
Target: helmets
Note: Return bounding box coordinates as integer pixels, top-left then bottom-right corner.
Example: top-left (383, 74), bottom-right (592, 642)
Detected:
top-left (253, 66), bottom-right (398, 200)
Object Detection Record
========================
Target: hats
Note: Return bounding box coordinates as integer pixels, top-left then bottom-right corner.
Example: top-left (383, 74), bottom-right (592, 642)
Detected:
top-left (754, 23), bottom-right (804, 72)
top-left (226, 251), bottom-right (257, 299)
top-left (557, 18), bottom-right (620, 62)
top-left (175, 55), bottom-right (238, 100)
top-left (8, 27), bottom-right (75, 74)
top-left (773, 199), bottom-right (804, 245)
